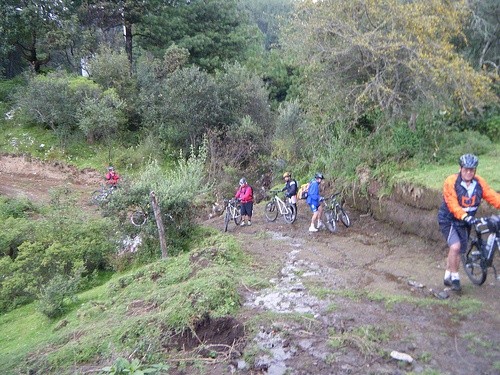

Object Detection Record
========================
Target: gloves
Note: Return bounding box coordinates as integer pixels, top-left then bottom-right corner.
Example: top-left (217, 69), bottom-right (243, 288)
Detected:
top-left (463, 216), bottom-right (479, 225)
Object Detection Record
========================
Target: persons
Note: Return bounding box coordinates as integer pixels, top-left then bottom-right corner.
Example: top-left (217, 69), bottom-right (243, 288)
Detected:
top-left (278, 172), bottom-right (298, 220)
top-left (307, 174), bottom-right (327, 232)
top-left (232, 178), bottom-right (254, 226)
top-left (106, 167), bottom-right (119, 188)
top-left (438, 154), bottom-right (499, 291)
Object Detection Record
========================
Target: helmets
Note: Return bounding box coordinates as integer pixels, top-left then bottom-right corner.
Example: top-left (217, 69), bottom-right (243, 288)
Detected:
top-left (315, 174), bottom-right (324, 180)
top-left (459, 154), bottom-right (479, 168)
top-left (239, 178), bottom-right (247, 185)
top-left (283, 172), bottom-right (290, 178)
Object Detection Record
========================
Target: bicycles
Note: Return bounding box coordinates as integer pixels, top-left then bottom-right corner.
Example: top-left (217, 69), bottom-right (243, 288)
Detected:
top-left (223, 199), bottom-right (243, 232)
top-left (91, 185), bottom-right (116, 203)
top-left (131, 202), bottom-right (172, 227)
top-left (264, 190), bottom-right (296, 224)
top-left (319, 192), bottom-right (350, 233)
top-left (461, 216), bottom-right (500, 286)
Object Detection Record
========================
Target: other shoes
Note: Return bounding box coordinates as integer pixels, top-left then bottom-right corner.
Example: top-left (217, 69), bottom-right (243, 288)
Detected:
top-left (444, 277), bottom-right (452, 286)
top-left (309, 227), bottom-right (319, 232)
top-left (240, 222), bottom-right (245, 227)
top-left (452, 279), bottom-right (461, 291)
top-left (317, 223), bottom-right (323, 228)
top-left (248, 221), bottom-right (252, 225)
